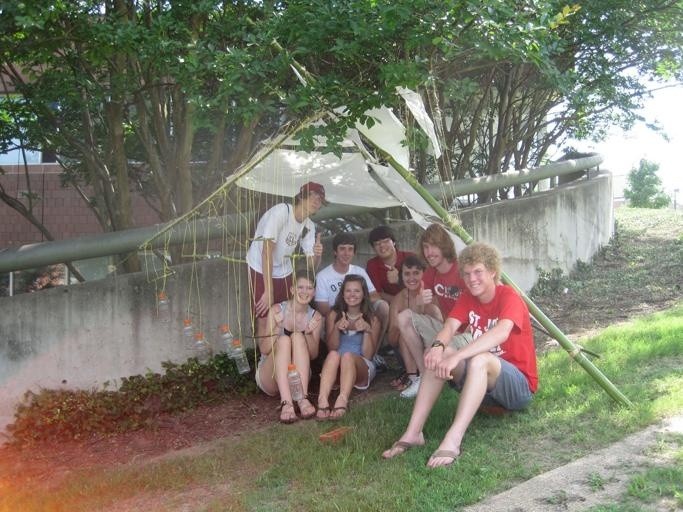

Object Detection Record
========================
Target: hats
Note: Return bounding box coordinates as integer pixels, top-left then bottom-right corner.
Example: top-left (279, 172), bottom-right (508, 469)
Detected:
top-left (301, 182), bottom-right (327, 204)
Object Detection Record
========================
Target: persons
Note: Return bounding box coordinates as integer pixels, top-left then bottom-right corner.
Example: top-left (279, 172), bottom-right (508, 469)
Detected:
top-left (314, 274), bottom-right (382, 421)
top-left (251, 271), bottom-right (323, 424)
top-left (365, 225), bottom-right (416, 303)
top-left (314, 232), bottom-right (389, 369)
top-left (243, 182), bottom-right (330, 364)
top-left (387, 256), bottom-right (444, 392)
top-left (379, 241), bottom-right (539, 471)
top-left (394, 224), bottom-right (466, 399)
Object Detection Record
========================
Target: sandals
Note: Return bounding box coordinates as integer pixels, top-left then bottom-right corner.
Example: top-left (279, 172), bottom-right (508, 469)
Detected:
top-left (280, 400), bottom-right (297, 424)
top-left (297, 395), bottom-right (316, 419)
top-left (389, 371), bottom-right (421, 398)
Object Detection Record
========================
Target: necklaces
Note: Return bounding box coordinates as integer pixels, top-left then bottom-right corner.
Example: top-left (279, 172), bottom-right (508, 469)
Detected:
top-left (345, 313), bottom-right (363, 320)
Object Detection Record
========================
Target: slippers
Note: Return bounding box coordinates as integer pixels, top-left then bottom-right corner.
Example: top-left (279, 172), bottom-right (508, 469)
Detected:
top-left (382, 439), bottom-right (428, 458)
top-left (329, 405), bottom-right (351, 420)
top-left (314, 406), bottom-right (332, 421)
top-left (426, 449), bottom-right (461, 468)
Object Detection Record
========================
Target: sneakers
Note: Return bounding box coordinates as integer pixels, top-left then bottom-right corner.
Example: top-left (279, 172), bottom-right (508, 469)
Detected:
top-left (374, 355), bottom-right (389, 372)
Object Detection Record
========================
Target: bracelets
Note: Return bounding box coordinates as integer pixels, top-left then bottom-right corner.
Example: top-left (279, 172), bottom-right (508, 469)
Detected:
top-left (430, 340), bottom-right (444, 351)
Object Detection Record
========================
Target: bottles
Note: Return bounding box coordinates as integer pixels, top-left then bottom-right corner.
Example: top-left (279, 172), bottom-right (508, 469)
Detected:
top-left (286, 364), bottom-right (304, 400)
top-left (232, 339), bottom-right (250, 376)
top-left (219, 324), bottom-right (233, 360)
top-left (158, 292), bottom-right (171, 324)
top-left (194, 333), bottom-right (209, 367)
top-left (183, 320), bottom-right (195, 350)
